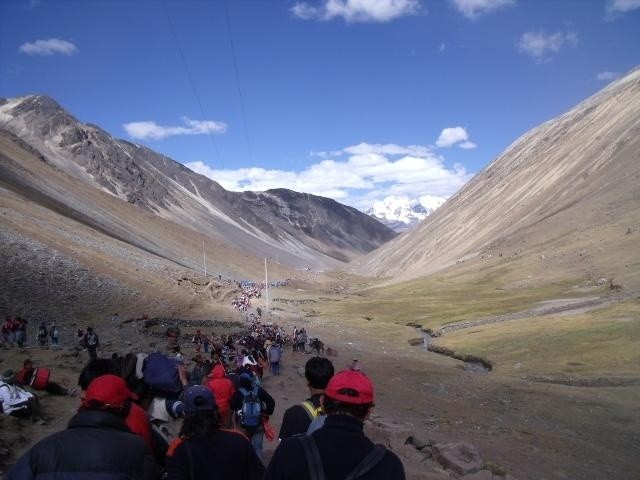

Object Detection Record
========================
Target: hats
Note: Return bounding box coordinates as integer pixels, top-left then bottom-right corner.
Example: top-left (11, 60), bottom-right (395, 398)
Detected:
top-left (183, 383), bottom-right (214, 413)
top-left (85, 374), bottom-right (139, 405)
top-left (239, 373), bottom-right (252, 384)
top-left (1, 368), bottom-right (17, 383)
top-left (325, 369), bottom-right (375, 405)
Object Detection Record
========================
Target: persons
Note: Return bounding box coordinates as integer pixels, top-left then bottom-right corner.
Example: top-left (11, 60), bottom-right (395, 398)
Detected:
top-left (157, 307), bottom-right (325, 384)
top-left (2, 349), bottom-right (405, 479)
top-left (2, 314), bottom-right (99, 360)
top-left (227, 279), bottom-right (289, 313)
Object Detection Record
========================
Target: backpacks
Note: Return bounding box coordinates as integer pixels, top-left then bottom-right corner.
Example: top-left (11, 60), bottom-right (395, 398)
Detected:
top-left (299, 400), bottom-right (328, 436)
top-left (237, 386), bottom-right (261, 429)
